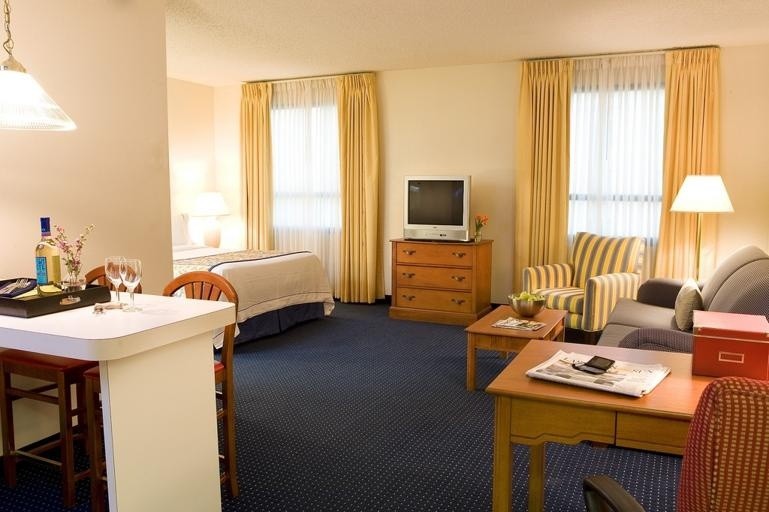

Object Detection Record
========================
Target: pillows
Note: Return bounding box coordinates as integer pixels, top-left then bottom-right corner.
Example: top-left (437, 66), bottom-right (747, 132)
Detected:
top-left (673, 276), bottom-right (702, 330)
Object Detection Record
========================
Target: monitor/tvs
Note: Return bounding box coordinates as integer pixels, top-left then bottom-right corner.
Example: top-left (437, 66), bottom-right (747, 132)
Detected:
top-left (403, 174), bottom-right (471, 242)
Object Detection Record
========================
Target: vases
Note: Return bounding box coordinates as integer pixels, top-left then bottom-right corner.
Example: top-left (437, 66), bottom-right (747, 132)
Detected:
top-left (475, 229), bottom-right (481, 240)
top-left (62, 262), bottom-right (86, 290)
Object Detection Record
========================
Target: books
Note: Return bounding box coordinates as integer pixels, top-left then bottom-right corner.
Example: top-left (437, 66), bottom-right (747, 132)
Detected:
top-left (491, 316), bottom-right (546, 332)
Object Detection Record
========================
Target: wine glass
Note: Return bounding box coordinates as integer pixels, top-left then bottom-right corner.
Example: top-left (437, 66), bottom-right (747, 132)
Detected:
top-left (119, 259), bottom-right (143, 313)
top-left (104, 255), bottom-right (126, 309)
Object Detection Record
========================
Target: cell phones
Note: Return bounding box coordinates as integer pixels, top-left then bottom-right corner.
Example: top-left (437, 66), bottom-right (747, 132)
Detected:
top-left (572, 355), bottom-right (615, 374)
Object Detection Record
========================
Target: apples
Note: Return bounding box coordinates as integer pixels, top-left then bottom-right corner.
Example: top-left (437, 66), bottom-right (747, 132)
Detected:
top-left (514, 289), bottom-right (544, 303)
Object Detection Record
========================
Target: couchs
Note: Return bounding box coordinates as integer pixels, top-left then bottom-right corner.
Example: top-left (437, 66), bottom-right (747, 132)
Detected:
top-left (523, 231), bottom-right (640, 335)
top-left (595, 245), bottom-right (769, 349)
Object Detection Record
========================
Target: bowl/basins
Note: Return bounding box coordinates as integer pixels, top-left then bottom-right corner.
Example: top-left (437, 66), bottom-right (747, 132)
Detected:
top-left (507, 294), bottom-right (546, 317)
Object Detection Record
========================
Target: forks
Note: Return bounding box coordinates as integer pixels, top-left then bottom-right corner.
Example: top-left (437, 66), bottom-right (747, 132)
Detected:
top-left (0, 277), bottom-right (29, 295)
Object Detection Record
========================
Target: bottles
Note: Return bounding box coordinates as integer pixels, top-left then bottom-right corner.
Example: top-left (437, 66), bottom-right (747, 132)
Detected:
top-left (34, 216), bottom-right (63, 297)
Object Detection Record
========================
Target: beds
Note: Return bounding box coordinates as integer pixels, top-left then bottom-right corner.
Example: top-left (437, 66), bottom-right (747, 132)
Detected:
top-left (171, 213), bottom-right (325, 344)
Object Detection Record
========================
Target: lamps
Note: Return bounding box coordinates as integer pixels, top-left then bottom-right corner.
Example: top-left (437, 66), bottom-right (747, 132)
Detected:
top-left (669, 174), bottom-right (734, 285)
top-left (1, 4), bottom-right (77, 139)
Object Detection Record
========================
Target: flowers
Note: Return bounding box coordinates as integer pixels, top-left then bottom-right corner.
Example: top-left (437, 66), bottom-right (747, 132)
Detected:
top-left (474, 215), bottom-right (488, 239)
top-left (50, 223), bottom-right (94, 288)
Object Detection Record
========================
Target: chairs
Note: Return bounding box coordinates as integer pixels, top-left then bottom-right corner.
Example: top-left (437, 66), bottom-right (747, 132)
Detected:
top-left (82, 272), bottom-right (244, 501)
top-left (0, 261), bottom-right (142, 506)
top-left (579, 374), bottom-right (768, 511)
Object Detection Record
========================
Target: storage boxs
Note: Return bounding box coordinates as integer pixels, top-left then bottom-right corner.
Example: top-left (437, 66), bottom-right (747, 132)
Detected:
top-left (692, 310), bottom-right (769, 379)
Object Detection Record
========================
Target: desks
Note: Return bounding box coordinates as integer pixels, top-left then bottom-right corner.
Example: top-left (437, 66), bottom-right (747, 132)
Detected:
top-left (485, 338), bottom-right (716, 512)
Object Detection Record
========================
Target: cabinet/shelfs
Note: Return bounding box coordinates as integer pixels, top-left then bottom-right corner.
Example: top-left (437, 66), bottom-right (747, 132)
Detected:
top-left (388, 239), bottom-right (496, 323)
